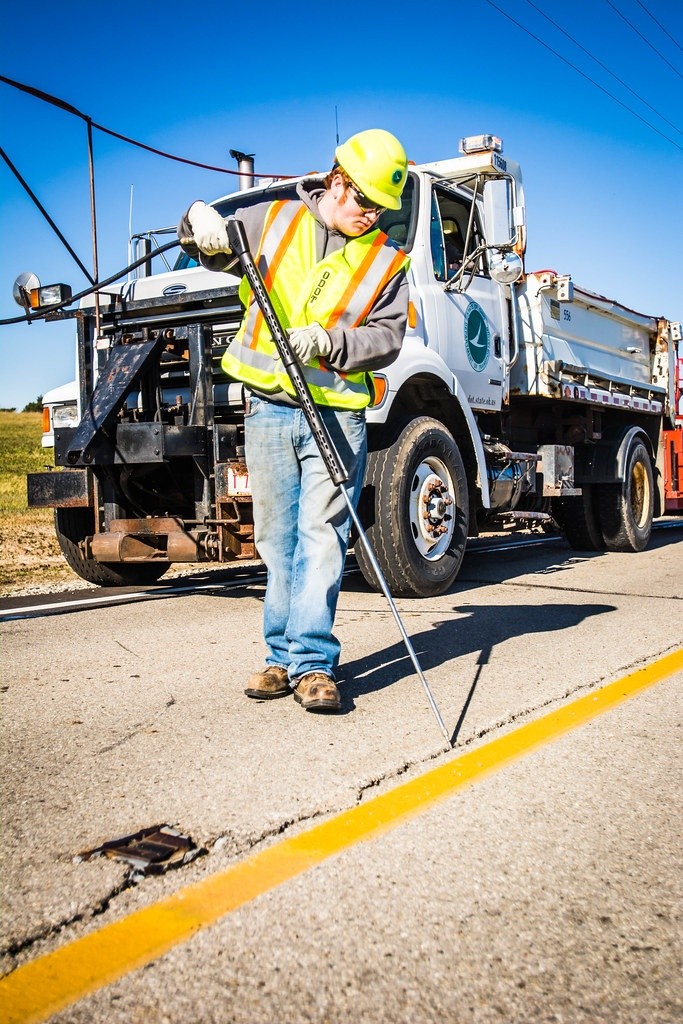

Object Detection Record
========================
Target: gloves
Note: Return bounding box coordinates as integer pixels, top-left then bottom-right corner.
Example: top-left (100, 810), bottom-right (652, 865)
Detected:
top-left (270, 324), bottom-right (331, 366)
top-left (187, 200), bottom-right (232, 255)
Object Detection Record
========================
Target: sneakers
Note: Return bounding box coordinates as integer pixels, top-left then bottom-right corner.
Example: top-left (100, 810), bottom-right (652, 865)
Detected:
top-left (294, 671), bottom-right (341, 710)
top-left (244, 663), bottom-right (290, 698)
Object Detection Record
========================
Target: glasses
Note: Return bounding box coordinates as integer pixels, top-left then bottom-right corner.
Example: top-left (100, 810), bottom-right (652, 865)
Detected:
top-left (345, 178), bottom-right (390, 216)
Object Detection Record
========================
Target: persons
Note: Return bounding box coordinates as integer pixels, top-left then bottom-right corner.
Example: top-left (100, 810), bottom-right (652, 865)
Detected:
top-left (175, 127), bottom-right (417, 707)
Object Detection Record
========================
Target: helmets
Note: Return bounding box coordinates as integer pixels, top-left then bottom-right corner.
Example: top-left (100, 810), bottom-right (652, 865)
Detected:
top-left (334, 128), bottom-right (409, 213)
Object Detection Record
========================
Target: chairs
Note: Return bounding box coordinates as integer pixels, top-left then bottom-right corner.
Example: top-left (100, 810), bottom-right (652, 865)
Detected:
top-left (443, 218), bottom-right (465, 266)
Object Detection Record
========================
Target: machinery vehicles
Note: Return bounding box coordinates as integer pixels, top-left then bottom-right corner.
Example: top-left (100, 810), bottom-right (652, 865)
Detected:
top-left (0, 134), bottom-right (683, 599)
